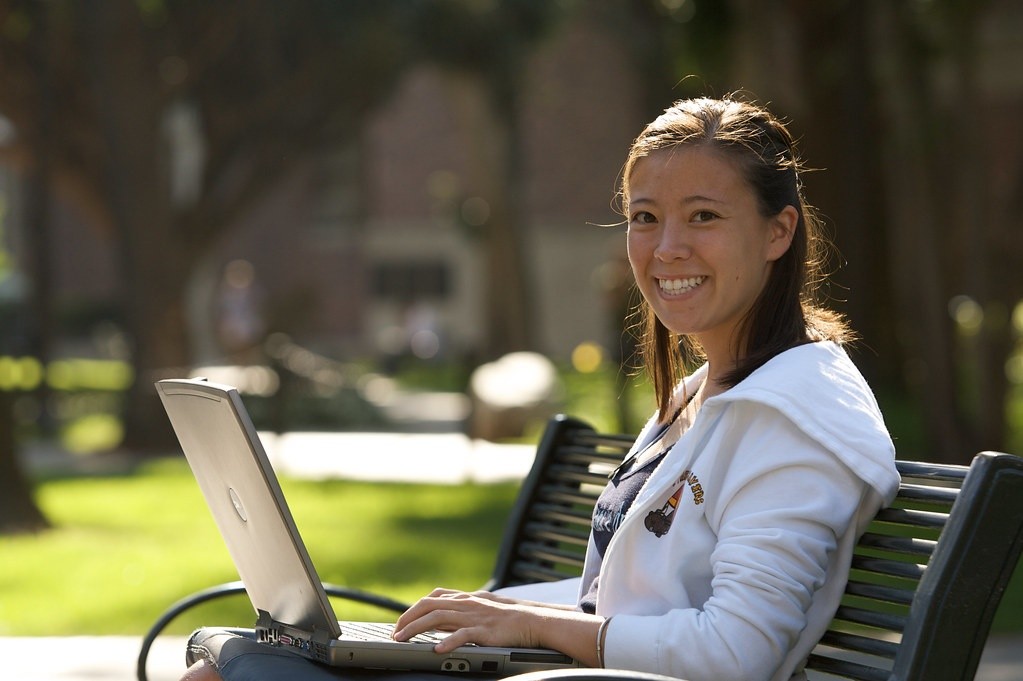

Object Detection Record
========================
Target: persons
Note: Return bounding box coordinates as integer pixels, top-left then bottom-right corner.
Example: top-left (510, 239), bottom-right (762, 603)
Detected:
top-left (178, 98), bottom-right (899, 681)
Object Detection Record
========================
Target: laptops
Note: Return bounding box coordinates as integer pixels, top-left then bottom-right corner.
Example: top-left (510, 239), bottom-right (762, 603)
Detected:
top-left (154, 377), bottom-right (580, 674)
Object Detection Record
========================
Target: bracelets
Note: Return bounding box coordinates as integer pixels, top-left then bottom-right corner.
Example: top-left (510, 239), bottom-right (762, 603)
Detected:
top-left (597, 617), bottom-right (612, 668)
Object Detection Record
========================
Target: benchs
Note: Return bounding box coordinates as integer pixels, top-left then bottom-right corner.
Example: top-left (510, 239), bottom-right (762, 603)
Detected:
top-left (137, 413), bottom-right (1023, 681)
